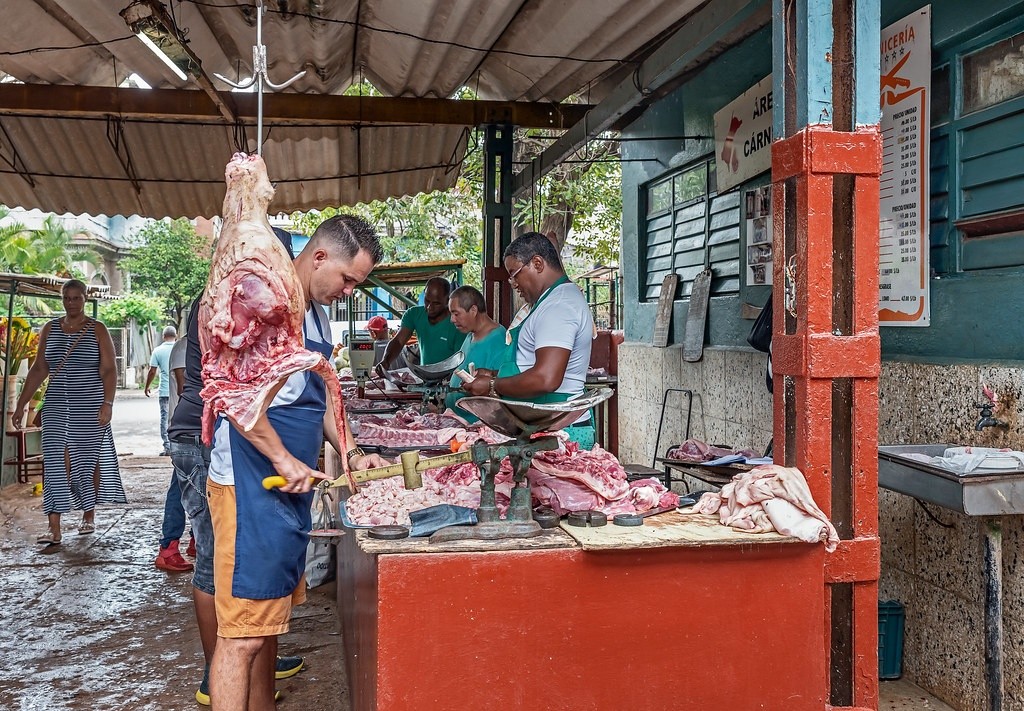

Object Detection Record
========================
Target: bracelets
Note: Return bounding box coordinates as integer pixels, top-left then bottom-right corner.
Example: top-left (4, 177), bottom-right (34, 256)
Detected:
top-left (104, 401), bottom-right (113, 406)
top-left (488, 377), bottom-right (501, 399)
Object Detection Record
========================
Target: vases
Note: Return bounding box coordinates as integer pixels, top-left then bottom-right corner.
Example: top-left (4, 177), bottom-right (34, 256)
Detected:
top-left (14, 399), bottom-right (42, 432)
top-left (0, 374), bottom-right (33, 433)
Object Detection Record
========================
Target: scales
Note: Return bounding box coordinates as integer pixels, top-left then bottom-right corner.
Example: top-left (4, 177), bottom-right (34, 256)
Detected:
top-left (348, 341), bottom-right (423, 400)
top-left (400, 351), bottom-right (465, 416)
top-left (308, 386), bottom-right (613, 544)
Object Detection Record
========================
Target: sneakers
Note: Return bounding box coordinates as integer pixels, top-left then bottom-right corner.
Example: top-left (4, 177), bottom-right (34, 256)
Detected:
top-left (155, 540), bottom-right (194, 572)
top-left (275, 655), bottom-right (303, 679)
top-left (186, 537), bottom-right (196, 556)
top-left (195, 671), bottom-right (280, 705)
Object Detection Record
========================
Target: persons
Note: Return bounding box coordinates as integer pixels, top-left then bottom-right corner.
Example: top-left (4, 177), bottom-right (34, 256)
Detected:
top-left (11, 280), bottom-right (128, 545)
top-left (364, 316), bottom-right (409, 371)
top-left (144, 325), bottom-right (177, 456)
top-left (460, 231), bottom-right (595, 452)
top-left (156, 226), bottom-right (306, 707)
top-left (443, 285), bottom-right (509, 425)
top-left (375, 278), bottom-right (466, 378)
top-left (206, 214), bottom-right (385, 711)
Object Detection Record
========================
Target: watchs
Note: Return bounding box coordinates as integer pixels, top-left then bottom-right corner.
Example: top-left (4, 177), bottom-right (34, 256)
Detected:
top-left (347, 447), bottom-right (366, 462)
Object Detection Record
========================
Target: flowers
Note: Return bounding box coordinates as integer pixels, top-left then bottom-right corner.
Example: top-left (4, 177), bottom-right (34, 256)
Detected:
top-left (0, 317), bottom-right (39, 376)
top-left (31, 483), bottom-right (43, 497)
top-left (19, 377), bottom-right (50, 401)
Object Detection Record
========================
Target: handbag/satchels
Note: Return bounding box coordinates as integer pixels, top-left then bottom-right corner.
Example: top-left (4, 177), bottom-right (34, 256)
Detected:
top-left (305, 487), bottom-right (337, 590)
top-left (32, 401), bottom-right (43, 428)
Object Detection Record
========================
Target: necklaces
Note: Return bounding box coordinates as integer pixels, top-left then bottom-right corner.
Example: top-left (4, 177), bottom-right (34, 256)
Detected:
top-left (63, 315), bottom-right (86, 328)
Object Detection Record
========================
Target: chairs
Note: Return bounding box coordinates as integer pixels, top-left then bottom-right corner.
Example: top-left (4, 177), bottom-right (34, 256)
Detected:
top-left (620, 388), bottom-right (692, 495)
top-left (372, 341), bottom-right (409, 369)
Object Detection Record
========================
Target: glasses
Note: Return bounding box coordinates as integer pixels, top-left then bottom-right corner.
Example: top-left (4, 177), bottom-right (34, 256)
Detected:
top-left (508, 255), bottom-right (535, 284)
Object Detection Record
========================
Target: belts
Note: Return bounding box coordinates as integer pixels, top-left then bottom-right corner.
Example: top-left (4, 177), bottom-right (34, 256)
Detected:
top-left (567, 418), bottom-right (592, 427)
top-left (172, 436), bottom-right (202, 445)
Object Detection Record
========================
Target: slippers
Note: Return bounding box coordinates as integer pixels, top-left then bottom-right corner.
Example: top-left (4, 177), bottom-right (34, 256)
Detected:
top-left (37, 533), bottom-right (61, 544)
top-left (77, 521), bottom-right (95, 535)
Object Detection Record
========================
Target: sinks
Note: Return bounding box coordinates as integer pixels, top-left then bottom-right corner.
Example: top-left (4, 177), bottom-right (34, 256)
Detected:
top-left (878, 443), bottom-right (1024, 516)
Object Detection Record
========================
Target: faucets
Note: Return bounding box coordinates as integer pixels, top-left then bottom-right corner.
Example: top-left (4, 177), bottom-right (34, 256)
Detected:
top-left (975, 403), bottom-right (998, 431)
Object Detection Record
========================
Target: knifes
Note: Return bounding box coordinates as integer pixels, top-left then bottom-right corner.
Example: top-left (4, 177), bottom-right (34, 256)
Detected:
top-left (262, 477), bottom-right (322, 491)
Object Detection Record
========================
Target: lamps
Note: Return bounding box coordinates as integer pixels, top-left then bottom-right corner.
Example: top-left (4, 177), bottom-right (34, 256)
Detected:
top-left (122, 2), bottom-right (202, 89)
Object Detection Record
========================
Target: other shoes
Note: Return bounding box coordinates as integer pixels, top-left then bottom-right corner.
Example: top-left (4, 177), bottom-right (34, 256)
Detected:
top-left (159, 449), bottom-right (171, 457)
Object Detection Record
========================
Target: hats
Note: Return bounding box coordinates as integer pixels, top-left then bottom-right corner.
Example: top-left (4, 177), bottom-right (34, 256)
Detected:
top-left (363, 316), bottom-right (388, 332)
